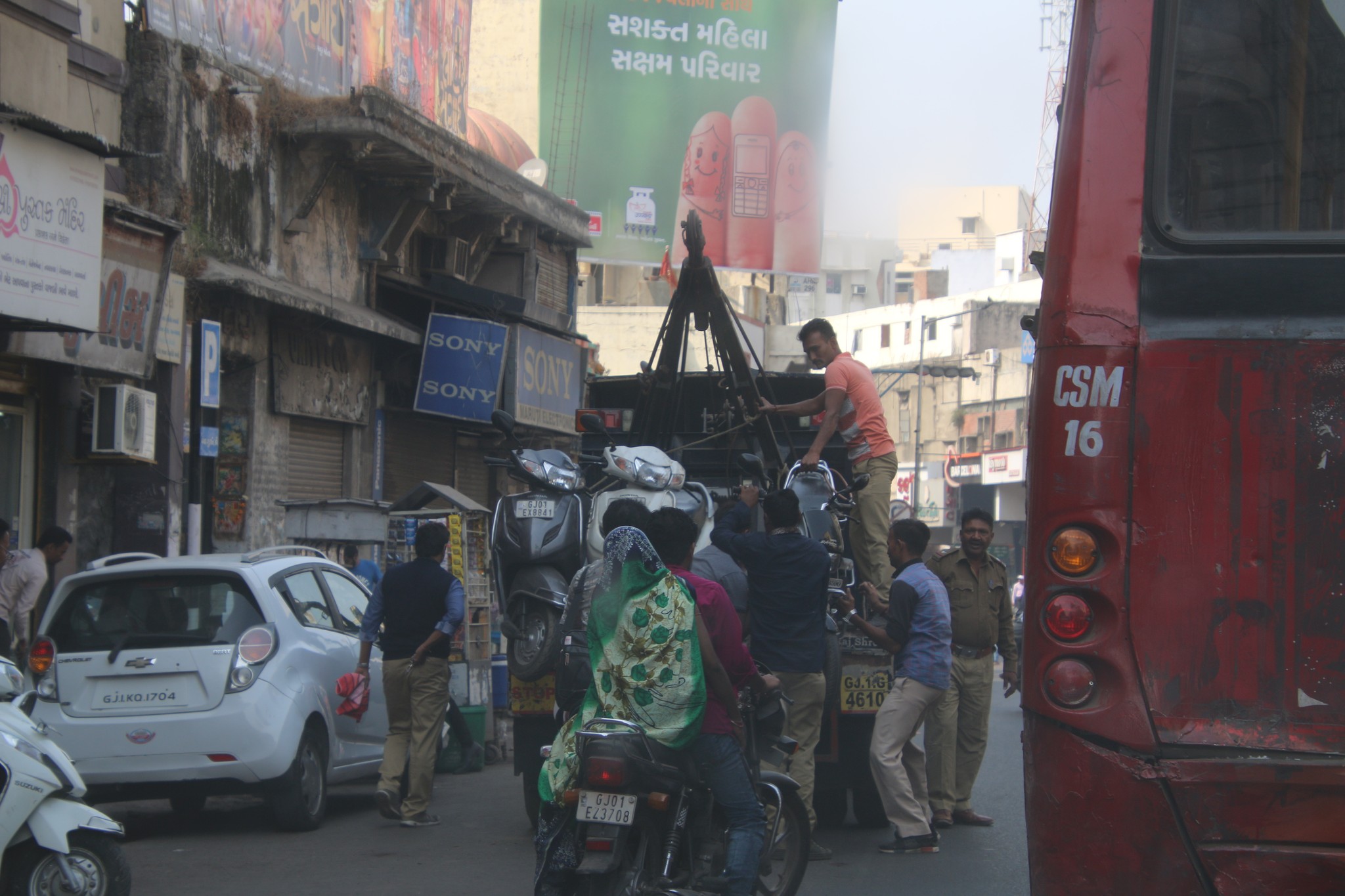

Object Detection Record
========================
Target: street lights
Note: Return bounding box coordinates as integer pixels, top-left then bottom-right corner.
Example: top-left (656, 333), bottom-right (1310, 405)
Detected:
top-left (911, 296), bottom-right (1004, 521)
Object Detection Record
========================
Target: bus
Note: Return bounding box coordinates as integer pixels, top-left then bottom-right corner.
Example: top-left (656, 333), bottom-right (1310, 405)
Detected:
top-left (1020, 1), bottom-right (1345, 896)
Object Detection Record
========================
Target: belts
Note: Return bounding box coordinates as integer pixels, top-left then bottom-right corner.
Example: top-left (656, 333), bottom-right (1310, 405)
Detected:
top-left (950, 642), bottom-right (992, 659)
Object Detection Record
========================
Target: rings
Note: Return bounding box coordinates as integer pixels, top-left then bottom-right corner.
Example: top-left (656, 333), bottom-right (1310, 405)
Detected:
top-left (870, 584), bottom-right (873, 587)
top-left (745, 486), bottom-right (749, 488)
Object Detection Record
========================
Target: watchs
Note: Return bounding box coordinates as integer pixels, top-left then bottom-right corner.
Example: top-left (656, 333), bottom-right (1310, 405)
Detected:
top-left (843, 608), bottom-right (857, 624)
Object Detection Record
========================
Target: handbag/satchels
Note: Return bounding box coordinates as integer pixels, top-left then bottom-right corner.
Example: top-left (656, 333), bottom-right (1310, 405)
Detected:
top-left (556, 629), bottom-right (594, 712)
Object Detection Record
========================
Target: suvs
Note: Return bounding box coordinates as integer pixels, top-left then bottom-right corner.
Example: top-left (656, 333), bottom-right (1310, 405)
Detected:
top-left (22, 544), bottom-right (450, 831)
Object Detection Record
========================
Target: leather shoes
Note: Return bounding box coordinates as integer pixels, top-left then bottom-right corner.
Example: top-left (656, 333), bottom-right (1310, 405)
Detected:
top-left (931, 809), bottom-right (953, 826)
top-left (951, 808), bottom-right (995, 825)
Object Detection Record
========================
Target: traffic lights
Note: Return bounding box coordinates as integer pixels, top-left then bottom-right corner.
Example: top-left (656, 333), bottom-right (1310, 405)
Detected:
top-left (915, 364), bottom-right (975, 379)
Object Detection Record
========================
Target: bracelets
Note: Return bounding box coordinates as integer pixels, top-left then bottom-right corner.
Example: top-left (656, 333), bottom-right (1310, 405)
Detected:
top-left (357, 662), bottom-right (369, 667)
top-left (772, 404), bottom-right (778, 413)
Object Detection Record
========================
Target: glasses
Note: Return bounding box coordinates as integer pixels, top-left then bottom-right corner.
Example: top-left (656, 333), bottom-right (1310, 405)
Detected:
top-left (0, 545), bottom-right (14, 560)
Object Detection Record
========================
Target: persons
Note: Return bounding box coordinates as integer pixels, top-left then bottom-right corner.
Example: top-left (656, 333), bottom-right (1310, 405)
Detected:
top-left (753, 316), bottom-right (901, 613)
top-left (537, 482), bottom-right (1024, 896)
top-left (0, 520), bottom-right (73, 663)
top-left (341, 523), bottom-right (466, 825)
top-left (674, 97), bottom-right (818, 275)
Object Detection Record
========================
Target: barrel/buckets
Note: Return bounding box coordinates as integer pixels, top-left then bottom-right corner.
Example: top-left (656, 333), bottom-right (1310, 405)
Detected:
top-left (434, 705), bottom-right (488, 774)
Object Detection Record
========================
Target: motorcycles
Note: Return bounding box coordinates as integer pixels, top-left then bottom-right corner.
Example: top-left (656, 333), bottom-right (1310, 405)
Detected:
top-left (575, 662), bottom-right (808, 896)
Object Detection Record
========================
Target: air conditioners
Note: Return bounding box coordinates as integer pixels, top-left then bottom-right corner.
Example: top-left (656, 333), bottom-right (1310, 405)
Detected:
top-left (434, 236), bottom-right (466, 281)
top-left (985, 348), bottom-right (999, 363)
top-left (92, 384), bottom-right (156, 460)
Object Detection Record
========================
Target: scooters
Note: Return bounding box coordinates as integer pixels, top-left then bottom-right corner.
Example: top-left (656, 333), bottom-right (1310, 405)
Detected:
top-left (1, 636), bottom-right (132, 896)
top-left (477, 403), bottom-right (852, 679)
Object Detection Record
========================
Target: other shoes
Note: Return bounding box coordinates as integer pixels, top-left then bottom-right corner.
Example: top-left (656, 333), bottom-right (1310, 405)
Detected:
top-left (770, 833), bottom-right (831, 859)
top-left (401, 809), bottom-right (441, 826)
top-left (375, 788), bottom-right (401, 819)
top-left (453, 741), bottom-right (484, 774)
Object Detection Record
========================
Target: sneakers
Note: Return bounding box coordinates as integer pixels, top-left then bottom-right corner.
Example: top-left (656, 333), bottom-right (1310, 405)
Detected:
top-left (894, 823), bottom-right (940, 839)
top-left (866, 607), bottom-right (888, 627)
top-left (877, 835), bottom-right (939, 853)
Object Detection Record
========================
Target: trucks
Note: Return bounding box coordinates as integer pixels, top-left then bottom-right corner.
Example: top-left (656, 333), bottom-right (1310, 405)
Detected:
top-left (493, 210), bottom-right (895, 828)
